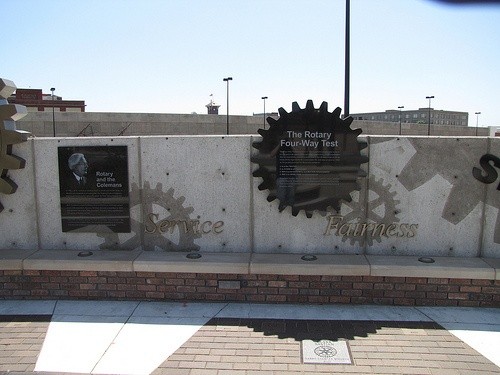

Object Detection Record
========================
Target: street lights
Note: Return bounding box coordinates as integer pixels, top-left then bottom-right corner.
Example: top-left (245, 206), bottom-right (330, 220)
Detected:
top-left (223, 77), bottom-right (232, 135)
top-left (398, 106), bottom-right (404, 135)
top-left (50, 87), bottom-right (55, 136)
top-left (426, 95), bottom-right (434, 136)
top-left (475, 111), bottom-right (481, 136)
top-left (262, 96), bottom-right (268, 130)
